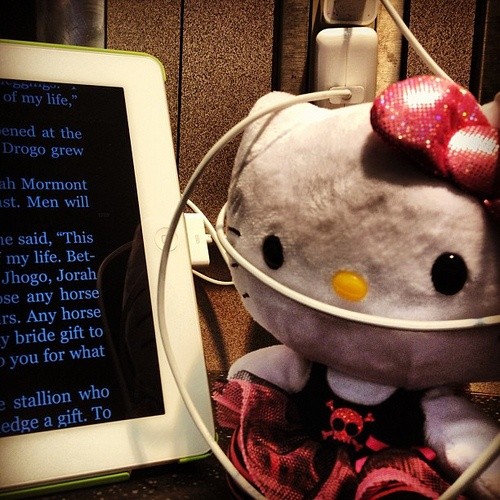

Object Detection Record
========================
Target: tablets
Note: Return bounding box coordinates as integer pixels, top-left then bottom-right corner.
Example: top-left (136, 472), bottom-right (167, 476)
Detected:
top-left (1, 39), bottom-right (215, 489)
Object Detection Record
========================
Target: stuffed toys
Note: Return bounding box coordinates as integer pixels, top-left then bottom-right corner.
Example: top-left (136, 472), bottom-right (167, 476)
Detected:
top-left (210, 74), bottom-right (499, 500)
